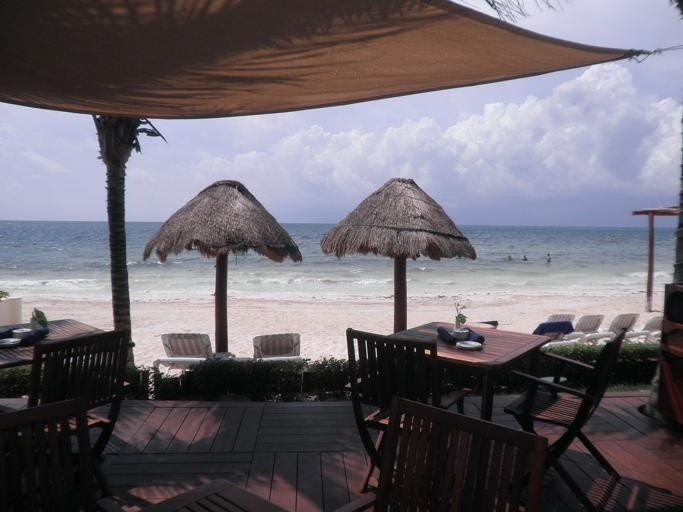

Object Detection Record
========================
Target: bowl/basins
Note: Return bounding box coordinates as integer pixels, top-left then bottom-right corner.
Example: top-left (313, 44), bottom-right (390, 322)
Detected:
top-left (11, 328), bottom-right (32, 338)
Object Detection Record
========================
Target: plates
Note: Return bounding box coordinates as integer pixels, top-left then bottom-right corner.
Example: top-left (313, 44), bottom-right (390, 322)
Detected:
top-left (0, 338), bottom-right (22, 347)
top-left (455, 340), bottom-right (481, 350)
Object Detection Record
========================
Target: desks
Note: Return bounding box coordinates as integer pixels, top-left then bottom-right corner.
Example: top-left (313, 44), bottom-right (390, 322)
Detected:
top-left (131, 475), bottom-right (296, 511)
top-left (371, 320), bottom-right (552, 462)
top-left (0, 314), bottom-right (135, 471)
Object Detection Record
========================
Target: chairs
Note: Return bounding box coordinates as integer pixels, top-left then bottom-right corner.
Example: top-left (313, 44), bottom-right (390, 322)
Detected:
top-left (153, 332), bottom-right (216, 381)
top-left (535, 310), bottom-right (665, 349)
top-left (0, 325), bottom-right (133, 500)
top-left (509, 326), bottom-right (629, 509)
top-left (0, 393), bottom-right (121, 511)
top-left (252, 333), bottom-right (308, 382)
top-left (343, 325), bottom-right (464, 497)
top-left (340, 394), bottom-right (551, 512)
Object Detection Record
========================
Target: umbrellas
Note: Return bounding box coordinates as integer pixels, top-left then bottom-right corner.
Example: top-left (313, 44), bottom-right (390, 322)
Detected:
top-left (318, 178), bottom-right (477, 335)
top-left (143, 180), bottom-right (303, 353)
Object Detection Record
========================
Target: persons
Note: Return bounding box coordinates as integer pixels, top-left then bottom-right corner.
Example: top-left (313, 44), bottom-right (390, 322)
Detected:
top-left (520, 255), bottom-right (528, 261)
top-left (544, 252), bottom-right (552, 264)
